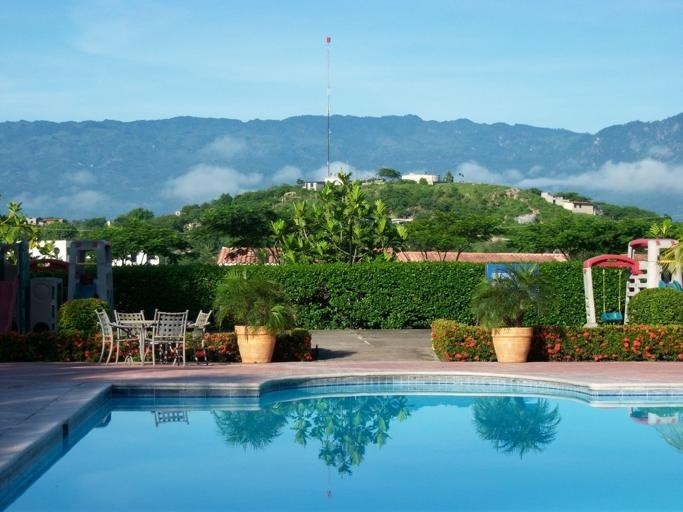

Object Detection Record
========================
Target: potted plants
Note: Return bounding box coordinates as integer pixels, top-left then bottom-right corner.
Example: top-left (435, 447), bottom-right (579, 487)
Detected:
top-left (468, 261), bottom-right (553, 363)
top-left (206, 263), bottom-right (296, 364)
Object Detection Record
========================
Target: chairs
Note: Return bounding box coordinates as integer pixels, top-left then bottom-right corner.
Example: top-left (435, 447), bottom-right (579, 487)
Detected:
top-left (94, 309), bottom-right (212, 368)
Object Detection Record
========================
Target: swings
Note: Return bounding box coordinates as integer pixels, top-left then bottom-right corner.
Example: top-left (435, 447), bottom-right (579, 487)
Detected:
top-left (601, 268), bottom-right (623, 322)
